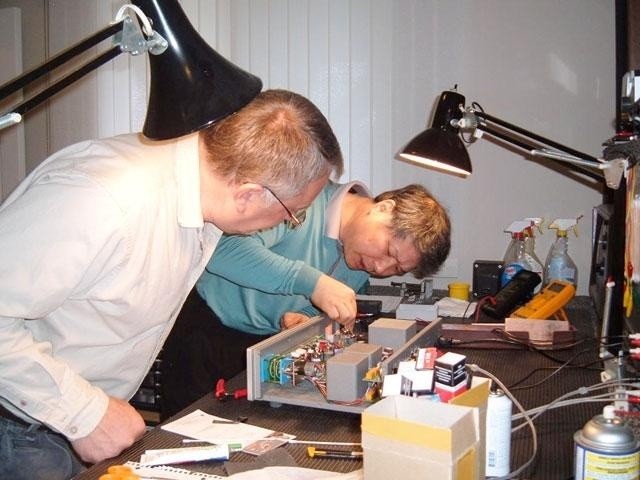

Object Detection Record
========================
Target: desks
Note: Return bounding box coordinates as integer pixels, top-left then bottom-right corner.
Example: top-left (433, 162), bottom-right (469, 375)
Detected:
top-left (63, 285), bottom-right (640, 480)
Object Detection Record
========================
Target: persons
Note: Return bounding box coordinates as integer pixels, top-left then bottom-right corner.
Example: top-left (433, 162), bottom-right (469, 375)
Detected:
top-left (0, 89), bottom-right (344, 478)
top-left (157, 180), bottom-right (451, 419)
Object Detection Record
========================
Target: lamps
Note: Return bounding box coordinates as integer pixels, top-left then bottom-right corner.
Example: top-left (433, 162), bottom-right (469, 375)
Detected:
top-left (396, 83), bottom-right (626, 191)
top-left (0, 0), bottom-right (264, 141)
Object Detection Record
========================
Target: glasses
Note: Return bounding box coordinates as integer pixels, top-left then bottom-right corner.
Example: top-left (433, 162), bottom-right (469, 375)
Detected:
top-left (243, 179), bottom-right (306, 229)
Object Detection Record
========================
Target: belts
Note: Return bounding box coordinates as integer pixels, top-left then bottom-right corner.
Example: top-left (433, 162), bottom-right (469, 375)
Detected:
top-left (0, 405), bottom-right (49, 435)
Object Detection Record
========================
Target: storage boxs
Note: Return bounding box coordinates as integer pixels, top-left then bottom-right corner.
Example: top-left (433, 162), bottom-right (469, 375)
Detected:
top-left (360, 395), bottom-right (482, 480)
top-left (449, 380), bottom-right (490, 480)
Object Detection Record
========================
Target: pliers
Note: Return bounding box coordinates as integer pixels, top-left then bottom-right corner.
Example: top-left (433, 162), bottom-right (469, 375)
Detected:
top-left (215, 379), bottom-right (247, 402)
